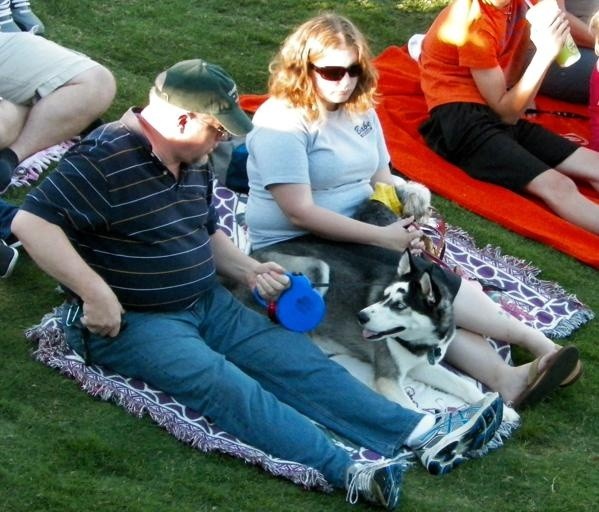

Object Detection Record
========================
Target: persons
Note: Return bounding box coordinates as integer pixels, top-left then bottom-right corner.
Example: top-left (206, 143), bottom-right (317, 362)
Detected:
top-left (9, 57), bottom-right (505, 511)
top-left (415, 0), bottom-right (599, 241)
top-left (558, 0), bottom-right (599, 48)
top-left (0, 0), bottom-right (118, 279)
top-left (242, 9), bottom-right (586, 415)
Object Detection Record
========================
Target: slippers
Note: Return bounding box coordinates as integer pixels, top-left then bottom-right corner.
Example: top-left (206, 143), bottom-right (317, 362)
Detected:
top-left (560, 361), bottom-right (584, 388)
top-left (508, 344), bottom-right (580, 414)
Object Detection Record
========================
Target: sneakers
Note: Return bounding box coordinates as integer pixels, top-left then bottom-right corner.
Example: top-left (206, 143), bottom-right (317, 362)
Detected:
top-left (347, 460), bottom-right (411, 509)
top-left (412, 389), bottom-right (504, 475)
top-left (0, 240), bottom-right (19, 278)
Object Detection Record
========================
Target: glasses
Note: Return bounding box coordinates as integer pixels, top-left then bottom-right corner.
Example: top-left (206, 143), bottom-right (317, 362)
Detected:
top-left (196, 115), bottom-right (234, 144)
top-left (309, 61), bottom-right (363, 82)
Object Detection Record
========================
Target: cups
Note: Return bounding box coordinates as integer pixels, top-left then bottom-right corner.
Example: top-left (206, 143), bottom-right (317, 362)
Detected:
top-left (525, 0), bottom-right (582, 70)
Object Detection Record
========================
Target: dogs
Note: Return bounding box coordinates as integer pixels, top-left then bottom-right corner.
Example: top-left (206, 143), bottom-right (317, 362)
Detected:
top-left (247, 229), bottom-right (520, 426)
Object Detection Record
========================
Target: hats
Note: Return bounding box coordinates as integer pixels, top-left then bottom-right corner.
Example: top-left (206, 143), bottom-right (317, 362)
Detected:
top-left (155, 58), bottom-right (255, 137)
